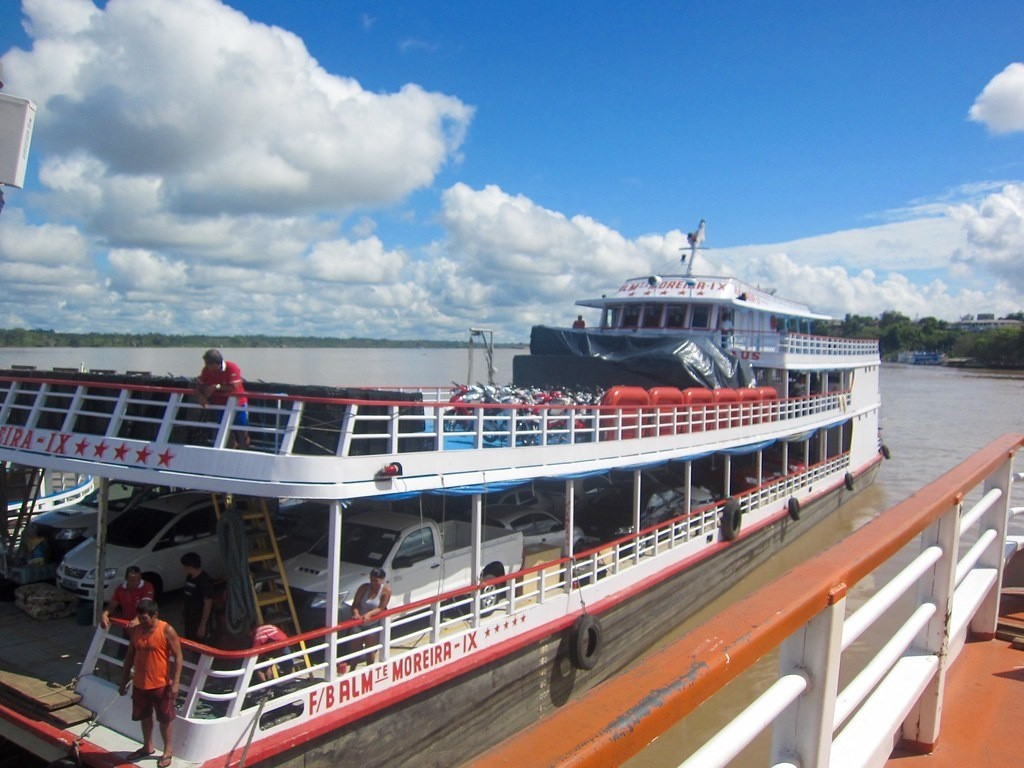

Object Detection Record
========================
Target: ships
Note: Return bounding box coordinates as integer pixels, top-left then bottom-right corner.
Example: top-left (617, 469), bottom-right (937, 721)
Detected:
top-left (0, 212), bottom-right (888, 768)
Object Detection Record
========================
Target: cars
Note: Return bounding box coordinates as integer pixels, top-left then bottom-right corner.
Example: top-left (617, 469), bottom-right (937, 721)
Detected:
top-left (16, 479), bottom-right (176, 574)
top-left (454, 505), bottom-right (586, 562)
top-left (490, 469), bottom-right (715, 561)
top-left (744, 449), bottom-right (805, 475)
top-left (669, 459), bottom-right (775, 497)
top-left (54, 492), bottom-right (273, 611)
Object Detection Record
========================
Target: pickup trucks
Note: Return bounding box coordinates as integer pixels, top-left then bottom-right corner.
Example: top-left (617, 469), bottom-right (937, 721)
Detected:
top-left (269, 511), bottom-right (524, 650)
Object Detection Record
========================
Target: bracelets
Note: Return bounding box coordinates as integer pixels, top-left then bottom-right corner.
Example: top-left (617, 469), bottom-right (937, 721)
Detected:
top-left (216, 384), bottom-right (222, 391)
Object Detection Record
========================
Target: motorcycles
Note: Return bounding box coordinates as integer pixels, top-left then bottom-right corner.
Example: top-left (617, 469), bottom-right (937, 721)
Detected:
top-left (441, 379), bottom-right (607, 443)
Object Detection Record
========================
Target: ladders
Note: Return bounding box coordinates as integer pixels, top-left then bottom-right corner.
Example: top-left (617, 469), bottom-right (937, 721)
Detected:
top-left (211, 491), bottom-right (314, 682)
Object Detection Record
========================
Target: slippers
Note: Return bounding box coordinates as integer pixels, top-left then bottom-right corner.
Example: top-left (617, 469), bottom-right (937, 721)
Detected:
top-left (126, 745), bottom-right (155, 761)
top-left (157, 754), bottom-right (172, 768)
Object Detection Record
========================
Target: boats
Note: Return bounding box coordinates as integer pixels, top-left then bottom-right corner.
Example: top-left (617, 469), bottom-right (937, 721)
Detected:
top-left (595, 385), bottom-right (648, 439)
top-left (896, 349), bottom-right (945, 365)
top-left (708, 386), bottom-right (737, 429)
top-left (753, 386), bottom-right (778, 420)
top-left (678, 388), bottom-right (713, 431)
top-left (735, 387), bottom-right (760, 424)
top-left (646, 385), bottom-right (684, 437)
top-left (943, 357), bottom-right (968, 368)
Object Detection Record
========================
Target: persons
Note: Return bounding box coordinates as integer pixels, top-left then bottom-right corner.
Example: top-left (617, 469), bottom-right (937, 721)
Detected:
top-left (244, 624), bottom-right (296, 692)
top-left (117, 598), bottom-right (181, 768)
top-left (99, 566), bottom-right (154, 661)
top-left (178, 552), bottom-right (214, 660)
top-left (572, 315), bottom-right (585, 329)
top-left (348, 568), bottom-right (391, 672)
top-left (195, 348), bottom-right (250, 450)
top-left (719, 314), bottom-right (732, 328)
